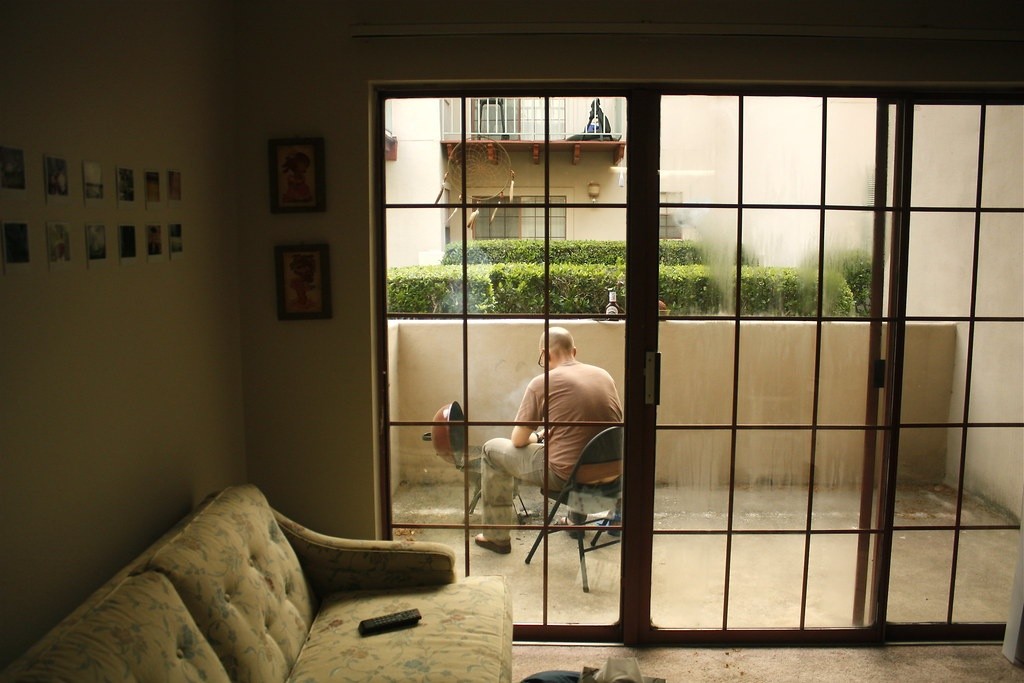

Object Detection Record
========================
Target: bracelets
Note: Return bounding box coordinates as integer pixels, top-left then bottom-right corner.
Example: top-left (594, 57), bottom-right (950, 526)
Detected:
top-left (534, 431), bottom-right (543, 442)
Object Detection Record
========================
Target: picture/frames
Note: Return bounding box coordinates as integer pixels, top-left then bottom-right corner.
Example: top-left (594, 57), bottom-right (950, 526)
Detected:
top-left (267, 137), bottom-right (326, 214)
top-left (274, 244), bottom-right (333, 322)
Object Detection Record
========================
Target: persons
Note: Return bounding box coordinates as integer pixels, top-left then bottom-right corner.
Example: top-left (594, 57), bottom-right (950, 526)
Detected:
top-left (473, 327), bottom-right (621, 555)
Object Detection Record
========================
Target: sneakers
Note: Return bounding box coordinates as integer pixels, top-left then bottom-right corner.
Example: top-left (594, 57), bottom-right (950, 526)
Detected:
top-left (561, 516), bottom-right (585, 539)
top-left (475, 533), bottom-right (511, 554)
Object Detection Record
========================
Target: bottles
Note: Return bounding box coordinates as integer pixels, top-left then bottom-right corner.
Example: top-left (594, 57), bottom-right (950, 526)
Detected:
top-left (606, 291), bottom-right (620, 320)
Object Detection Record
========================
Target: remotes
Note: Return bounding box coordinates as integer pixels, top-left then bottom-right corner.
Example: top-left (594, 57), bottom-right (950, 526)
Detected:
top-left (358, 608), bottom-right (422, 634)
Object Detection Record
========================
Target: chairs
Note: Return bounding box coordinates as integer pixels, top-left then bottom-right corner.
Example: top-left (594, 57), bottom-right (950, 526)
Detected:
top-left (524, 425), bottom-right (623, 593)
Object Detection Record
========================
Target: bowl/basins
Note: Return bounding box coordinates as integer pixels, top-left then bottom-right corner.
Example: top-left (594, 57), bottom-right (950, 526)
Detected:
top-left (659, 310), bottom-right (670, 316)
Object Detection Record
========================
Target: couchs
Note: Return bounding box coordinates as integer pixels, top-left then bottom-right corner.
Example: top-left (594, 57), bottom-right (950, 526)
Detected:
top-left (0, 482), bottom-right (512, 683)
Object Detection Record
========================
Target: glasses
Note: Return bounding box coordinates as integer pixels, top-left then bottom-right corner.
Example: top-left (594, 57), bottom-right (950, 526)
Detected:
top-left (538, 347), bottom-right (546, 368)
